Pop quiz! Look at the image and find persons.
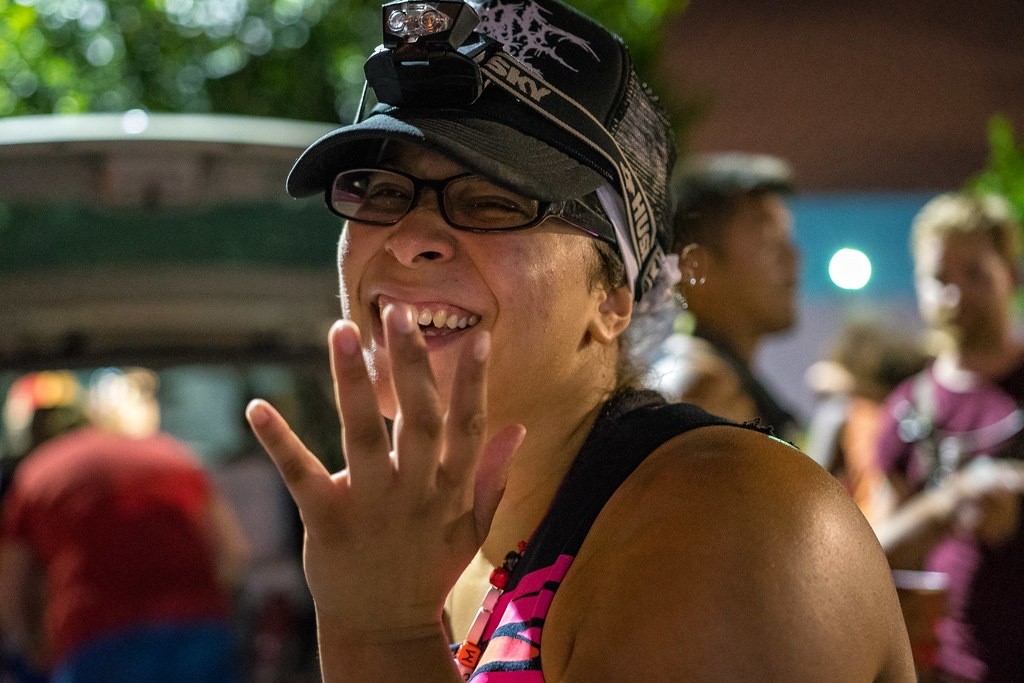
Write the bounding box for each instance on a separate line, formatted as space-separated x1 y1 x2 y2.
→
245 0 917 683
866 190 1024 683
644 151 804 443
0 405 251 683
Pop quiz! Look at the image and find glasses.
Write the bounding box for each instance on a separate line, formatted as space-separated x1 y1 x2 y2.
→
323 167 617 245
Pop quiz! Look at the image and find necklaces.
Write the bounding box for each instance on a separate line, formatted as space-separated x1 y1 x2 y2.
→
453 540 530 682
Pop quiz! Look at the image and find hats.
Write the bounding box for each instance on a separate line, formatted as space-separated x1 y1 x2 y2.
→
285 0 675 250
